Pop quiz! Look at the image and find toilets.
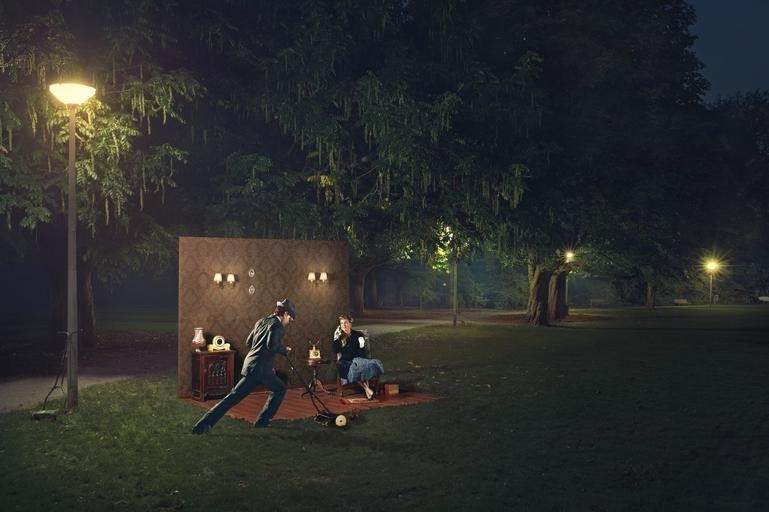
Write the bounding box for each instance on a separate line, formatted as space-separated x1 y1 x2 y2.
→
337 325 343 335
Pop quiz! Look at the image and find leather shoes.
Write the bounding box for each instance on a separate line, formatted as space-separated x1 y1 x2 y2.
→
333 325 342 341
358 337 365 349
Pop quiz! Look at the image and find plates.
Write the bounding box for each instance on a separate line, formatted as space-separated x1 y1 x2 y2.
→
193 349 236 401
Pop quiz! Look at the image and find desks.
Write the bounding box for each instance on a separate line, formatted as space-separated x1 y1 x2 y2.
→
705 259 719 311
49 79 98 410
565 252 573 305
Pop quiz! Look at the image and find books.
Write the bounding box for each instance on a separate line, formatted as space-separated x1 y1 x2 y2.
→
384 384 400 395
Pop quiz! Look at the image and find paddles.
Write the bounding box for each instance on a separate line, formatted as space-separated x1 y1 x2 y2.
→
181 382 442 424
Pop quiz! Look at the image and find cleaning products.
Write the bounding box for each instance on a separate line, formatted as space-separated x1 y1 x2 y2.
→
284 344 359 427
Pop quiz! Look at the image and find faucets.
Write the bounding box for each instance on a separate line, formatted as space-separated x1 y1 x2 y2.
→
365 387 374 400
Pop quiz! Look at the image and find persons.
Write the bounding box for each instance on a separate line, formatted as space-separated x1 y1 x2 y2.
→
191 298 297 435
332 313 385 401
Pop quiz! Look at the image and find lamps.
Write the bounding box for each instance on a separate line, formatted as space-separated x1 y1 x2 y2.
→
334 329 374 397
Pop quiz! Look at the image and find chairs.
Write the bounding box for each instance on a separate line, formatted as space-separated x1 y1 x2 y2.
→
255 419 272 428
192 424 205 434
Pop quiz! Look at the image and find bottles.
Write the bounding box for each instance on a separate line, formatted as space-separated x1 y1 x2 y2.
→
277 299 297 320
